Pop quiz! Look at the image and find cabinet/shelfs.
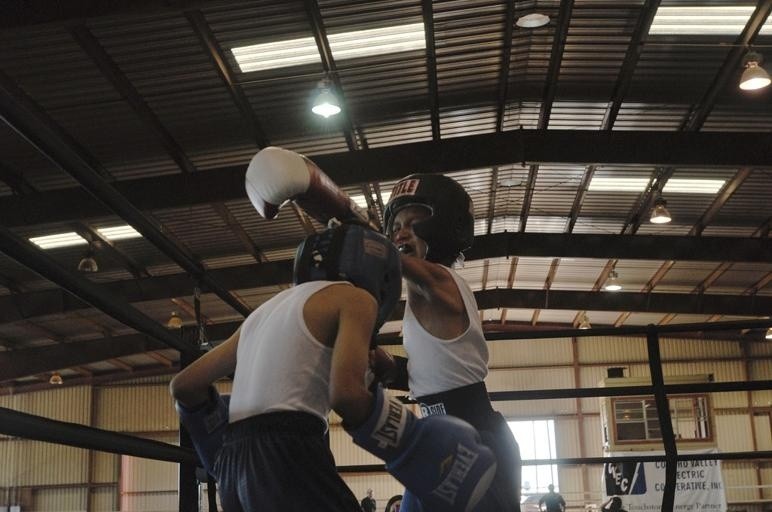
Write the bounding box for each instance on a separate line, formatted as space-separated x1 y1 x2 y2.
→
596 375 718 456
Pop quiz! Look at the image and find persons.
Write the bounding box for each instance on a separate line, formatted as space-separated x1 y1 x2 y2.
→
539 483 566 511
384 495 403 512
360 488 377 511
600 495 627 511
170 220 510 511
244 143 522 512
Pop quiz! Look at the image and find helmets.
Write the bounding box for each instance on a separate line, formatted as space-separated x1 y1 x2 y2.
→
291 223 402 350
382 172 475 267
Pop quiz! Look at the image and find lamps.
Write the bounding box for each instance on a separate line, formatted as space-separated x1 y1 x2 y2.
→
604 267 622 293
78 239 100 272
645 184 673 227
50 365 64 383
578 313 591 331
311 70 343 121
168 303 182 328
738 52 771 91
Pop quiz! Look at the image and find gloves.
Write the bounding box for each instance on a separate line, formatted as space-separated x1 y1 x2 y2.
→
244 145 370 228
340 381 498 511
174 387 229 480
